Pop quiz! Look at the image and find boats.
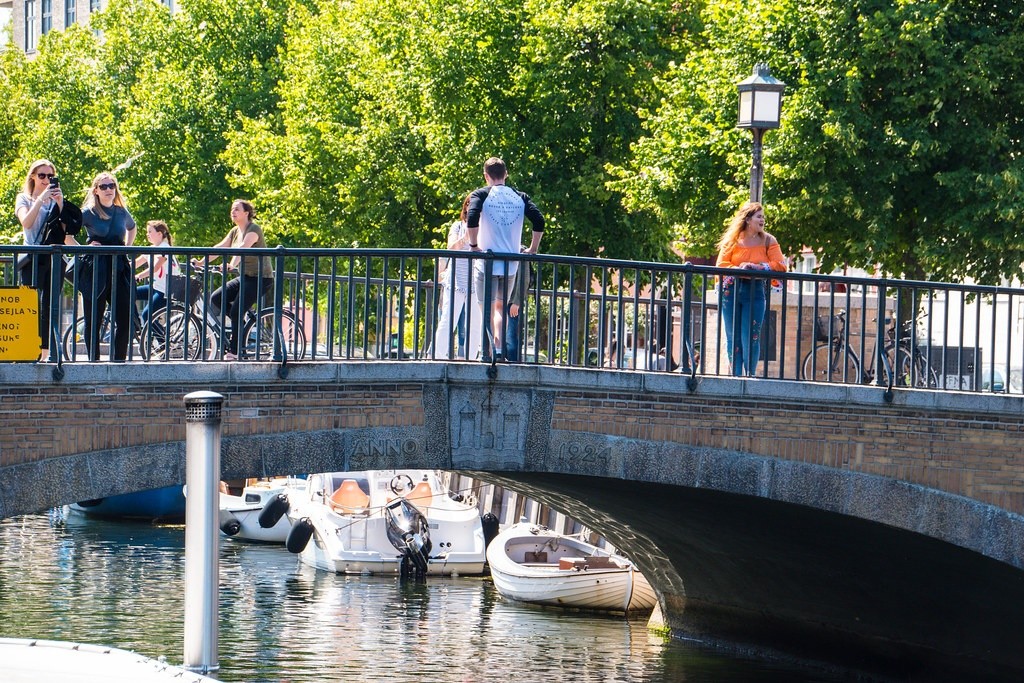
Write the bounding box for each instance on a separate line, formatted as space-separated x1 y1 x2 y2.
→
218 478 307 541
485 516 658 613
69 485 187 517
280 468 486 577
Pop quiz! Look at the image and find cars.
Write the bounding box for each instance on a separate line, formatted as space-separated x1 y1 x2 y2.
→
376 349 426 359
283 342 327 356
520 348 546 363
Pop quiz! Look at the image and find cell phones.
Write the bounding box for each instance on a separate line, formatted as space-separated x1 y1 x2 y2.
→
50 178 58 189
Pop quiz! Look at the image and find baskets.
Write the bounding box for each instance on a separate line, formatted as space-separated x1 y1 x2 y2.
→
817 314 838 341
887 326 921 347
166 273 202 303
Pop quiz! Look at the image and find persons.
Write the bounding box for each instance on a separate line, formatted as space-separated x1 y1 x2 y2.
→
64 172 136 362
426 157 546 363
129 221 182 361
190 198 274 361
603 337 699 375
715 201 786 378
15 159 66 363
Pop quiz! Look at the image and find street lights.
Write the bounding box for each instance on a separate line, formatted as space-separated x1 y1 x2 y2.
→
735 62 787 205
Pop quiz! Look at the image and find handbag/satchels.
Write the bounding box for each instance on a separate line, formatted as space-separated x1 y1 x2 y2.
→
65 254 106 300
14 253 31 272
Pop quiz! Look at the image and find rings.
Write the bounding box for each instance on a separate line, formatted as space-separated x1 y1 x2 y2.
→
47 190 49 192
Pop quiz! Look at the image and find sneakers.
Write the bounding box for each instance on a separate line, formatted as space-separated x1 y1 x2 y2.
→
103 335 112 342
224 353 238 360
492 348 505 361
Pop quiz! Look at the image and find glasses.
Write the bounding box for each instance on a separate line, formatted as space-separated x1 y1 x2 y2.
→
98 183 115 190
37 173 55 180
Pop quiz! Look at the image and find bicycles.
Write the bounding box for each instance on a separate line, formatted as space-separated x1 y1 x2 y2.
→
63 278 218 363
139 262 306 362
884 314 939 389
802 311 919 389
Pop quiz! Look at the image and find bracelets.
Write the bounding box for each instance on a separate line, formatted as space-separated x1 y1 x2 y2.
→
36 195 45 203
469 243 478 248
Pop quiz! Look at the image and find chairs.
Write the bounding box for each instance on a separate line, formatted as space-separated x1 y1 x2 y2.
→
331 479 370 516
387 482 433 514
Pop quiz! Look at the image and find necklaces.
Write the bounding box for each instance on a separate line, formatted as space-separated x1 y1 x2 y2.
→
32 196 49 205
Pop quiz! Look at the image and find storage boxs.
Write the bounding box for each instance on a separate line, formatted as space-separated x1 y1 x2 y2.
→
559 557 586 570
